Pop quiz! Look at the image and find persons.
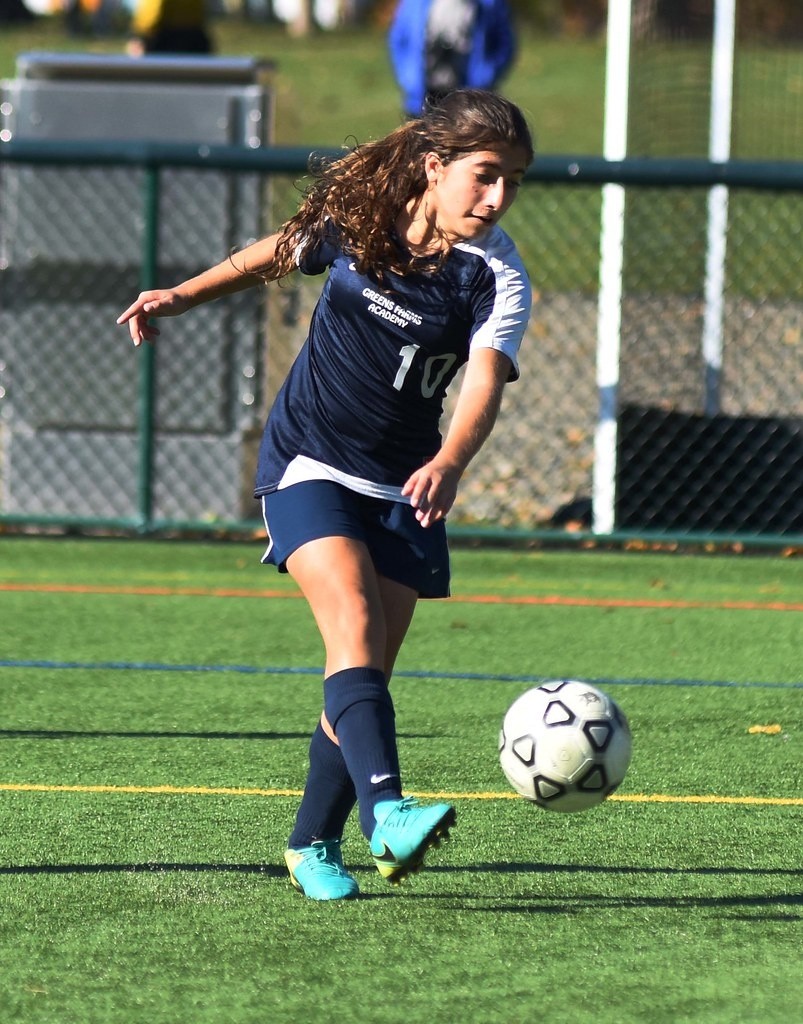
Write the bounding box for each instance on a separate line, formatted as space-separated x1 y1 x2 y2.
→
64 0 365 58
115 90 534 904
388 0 516 118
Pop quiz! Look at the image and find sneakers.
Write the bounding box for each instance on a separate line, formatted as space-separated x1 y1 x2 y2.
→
369 796 457 888
284 837 359 901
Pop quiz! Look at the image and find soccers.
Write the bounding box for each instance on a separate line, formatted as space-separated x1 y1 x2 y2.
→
498 679 635 814
